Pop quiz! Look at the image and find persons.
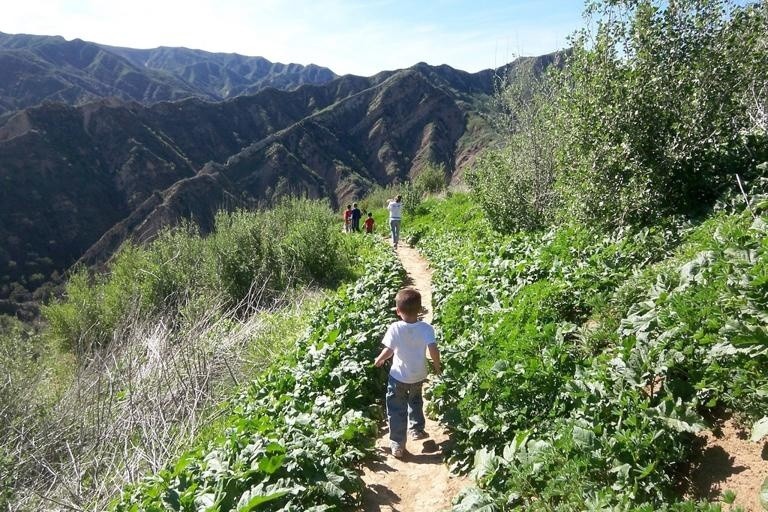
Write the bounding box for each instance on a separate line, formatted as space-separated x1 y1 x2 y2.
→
348 204 361 233
361 212 375 234
344 204 352 233
373 289 442 460
387 195 402 250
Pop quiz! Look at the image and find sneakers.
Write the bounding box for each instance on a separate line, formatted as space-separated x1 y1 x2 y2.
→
411 428 430 441
390 439 406 458
394 243 397 250
